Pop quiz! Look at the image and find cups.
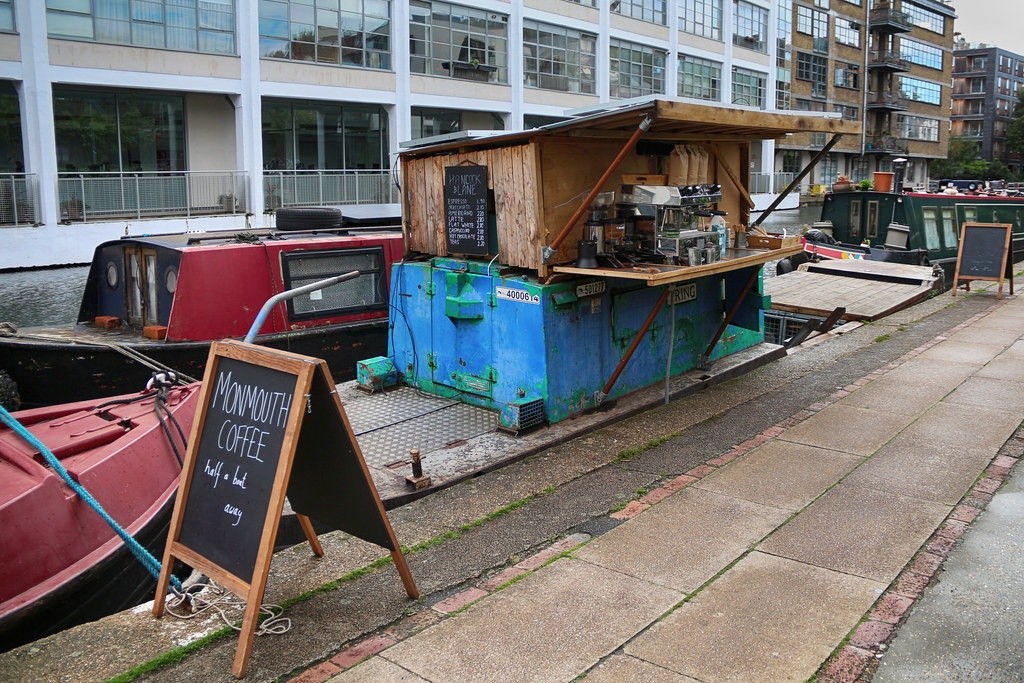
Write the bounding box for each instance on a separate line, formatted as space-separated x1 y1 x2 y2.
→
688 246 702 266
734 232 750 248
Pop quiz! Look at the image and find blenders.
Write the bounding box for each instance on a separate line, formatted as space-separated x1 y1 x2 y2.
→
582 191 624 256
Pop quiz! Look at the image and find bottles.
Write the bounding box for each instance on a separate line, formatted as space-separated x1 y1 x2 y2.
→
706 249 716 264
708 215 725 232
859 242 870 248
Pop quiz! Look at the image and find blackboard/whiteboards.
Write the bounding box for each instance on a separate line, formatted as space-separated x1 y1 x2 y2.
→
149 337 398 597
952 221 1014 282
443 164 490 258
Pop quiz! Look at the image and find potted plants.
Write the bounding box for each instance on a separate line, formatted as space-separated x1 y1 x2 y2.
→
265 183 280 206
860 179 873 191
217 193 237 210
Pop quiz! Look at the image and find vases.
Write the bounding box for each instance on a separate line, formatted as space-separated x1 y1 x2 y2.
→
872 172 895 192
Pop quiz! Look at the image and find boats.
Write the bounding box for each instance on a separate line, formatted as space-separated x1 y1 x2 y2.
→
0 206 404 415
777 157 1024 290
0 379 205 655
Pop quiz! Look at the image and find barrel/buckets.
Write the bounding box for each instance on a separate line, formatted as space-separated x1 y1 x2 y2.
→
812 220 833 239
884 223 911 249
872 171 895 192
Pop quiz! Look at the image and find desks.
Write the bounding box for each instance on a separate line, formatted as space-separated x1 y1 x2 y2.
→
441 61 498 82
